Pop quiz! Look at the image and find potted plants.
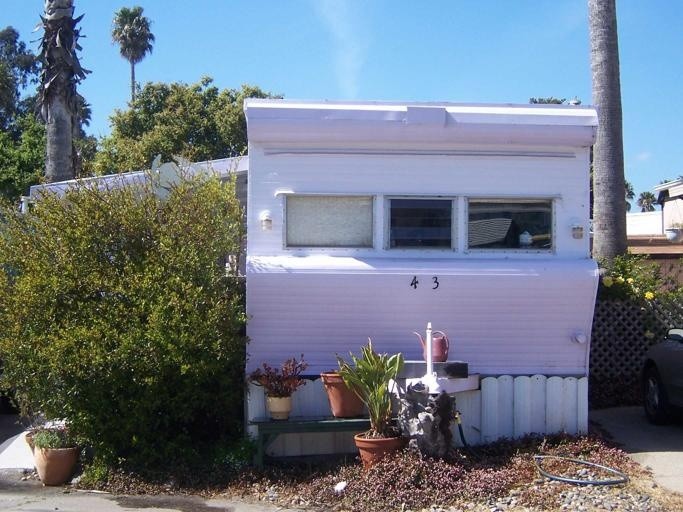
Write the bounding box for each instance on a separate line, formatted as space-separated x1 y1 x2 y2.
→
25 417 60 452
335 335 403 466
34 430 78 486
245 353 309 420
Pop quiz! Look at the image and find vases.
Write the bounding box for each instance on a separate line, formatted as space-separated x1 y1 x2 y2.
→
320 372 364 418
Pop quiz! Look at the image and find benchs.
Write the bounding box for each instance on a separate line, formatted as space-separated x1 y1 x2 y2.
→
249 416 399 471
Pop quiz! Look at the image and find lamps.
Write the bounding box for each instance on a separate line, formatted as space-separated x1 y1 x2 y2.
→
261 211 272 232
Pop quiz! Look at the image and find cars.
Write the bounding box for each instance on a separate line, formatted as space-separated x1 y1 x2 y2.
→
642 329 682 425
